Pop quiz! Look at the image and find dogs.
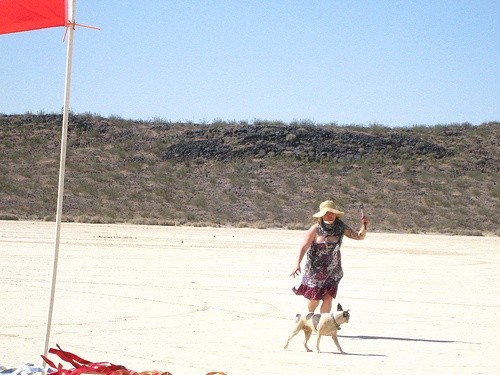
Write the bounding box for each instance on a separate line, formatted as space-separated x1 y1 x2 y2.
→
283 304 352 354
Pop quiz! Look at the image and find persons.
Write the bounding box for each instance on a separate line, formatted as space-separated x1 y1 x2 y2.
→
289 201 369 334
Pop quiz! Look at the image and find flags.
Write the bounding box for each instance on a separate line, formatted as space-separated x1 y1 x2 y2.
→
0 0 71 36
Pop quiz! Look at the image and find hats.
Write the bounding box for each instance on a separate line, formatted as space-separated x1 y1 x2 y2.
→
312 200 344 218
